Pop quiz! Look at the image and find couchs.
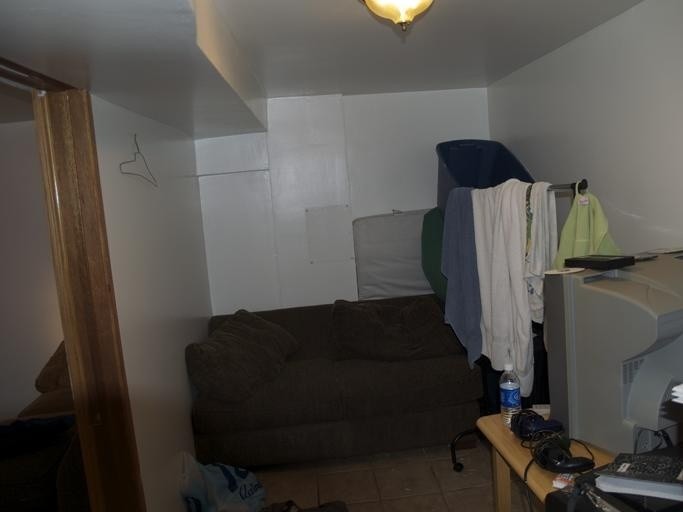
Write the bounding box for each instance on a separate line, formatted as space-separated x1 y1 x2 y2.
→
191 294 484 469
0 342 90 510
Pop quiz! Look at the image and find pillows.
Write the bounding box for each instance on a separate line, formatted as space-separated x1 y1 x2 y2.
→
329 300 450 361
185 309 300 403
0 414 75 460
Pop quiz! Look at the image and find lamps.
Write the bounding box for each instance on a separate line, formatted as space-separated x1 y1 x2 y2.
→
357 1 435 31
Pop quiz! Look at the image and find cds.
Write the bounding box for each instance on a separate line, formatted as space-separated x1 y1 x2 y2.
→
545 267 584 274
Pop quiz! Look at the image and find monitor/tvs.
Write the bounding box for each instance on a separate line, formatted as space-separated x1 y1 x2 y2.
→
543 248 683 455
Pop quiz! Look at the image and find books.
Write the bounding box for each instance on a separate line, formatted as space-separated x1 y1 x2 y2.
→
592 382 683 501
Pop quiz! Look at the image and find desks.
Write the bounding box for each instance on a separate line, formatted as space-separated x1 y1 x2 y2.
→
476 411 614 512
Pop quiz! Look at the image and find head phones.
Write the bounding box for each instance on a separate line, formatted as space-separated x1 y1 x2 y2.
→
511 411 564 439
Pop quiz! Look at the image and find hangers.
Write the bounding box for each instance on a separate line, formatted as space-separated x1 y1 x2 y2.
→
121 135 158 188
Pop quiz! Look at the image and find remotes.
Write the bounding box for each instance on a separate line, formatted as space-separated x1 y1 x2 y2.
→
532 405 550 414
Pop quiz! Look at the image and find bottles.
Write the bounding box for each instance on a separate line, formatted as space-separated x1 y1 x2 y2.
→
499 363 522 426
